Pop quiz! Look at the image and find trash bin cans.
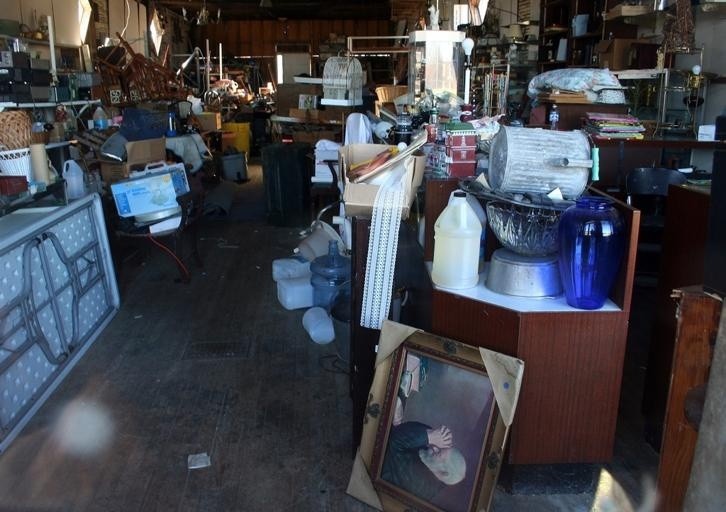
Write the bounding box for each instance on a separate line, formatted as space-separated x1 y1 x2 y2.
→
259 143 314 227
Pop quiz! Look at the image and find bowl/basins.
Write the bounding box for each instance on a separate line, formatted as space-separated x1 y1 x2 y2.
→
486 200 569 257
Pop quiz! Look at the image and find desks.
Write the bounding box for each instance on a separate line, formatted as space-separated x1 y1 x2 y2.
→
111 195 204 287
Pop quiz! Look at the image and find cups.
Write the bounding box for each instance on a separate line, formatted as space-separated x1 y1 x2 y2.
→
29 144 50 186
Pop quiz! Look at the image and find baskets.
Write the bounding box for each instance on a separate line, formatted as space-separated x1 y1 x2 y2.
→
1 110 35 150
374 84 409 103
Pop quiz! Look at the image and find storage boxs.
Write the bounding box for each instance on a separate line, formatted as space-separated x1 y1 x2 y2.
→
597 36 662 73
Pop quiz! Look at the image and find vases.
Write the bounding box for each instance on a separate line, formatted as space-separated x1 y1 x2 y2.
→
553 194 628 311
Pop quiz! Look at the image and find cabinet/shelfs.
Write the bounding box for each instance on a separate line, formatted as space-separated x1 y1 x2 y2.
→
0 31 101 177
535 0 586 82
0 188 123 458
470 39 538 126
628 178 715 390
339 209 425 462
406 29 470 106
529 95 667 210
404 168 646 502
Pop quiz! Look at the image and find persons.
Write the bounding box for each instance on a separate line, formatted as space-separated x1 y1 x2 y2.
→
377 413 472 504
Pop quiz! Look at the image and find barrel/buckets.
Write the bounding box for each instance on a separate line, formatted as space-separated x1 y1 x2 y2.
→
487 124 601 200
431 191 484 291
61 159 84 200
327 278 352 373
310 239 351 310
92 104 108 130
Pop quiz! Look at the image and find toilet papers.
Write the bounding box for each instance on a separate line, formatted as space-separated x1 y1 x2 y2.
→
29 143 60 188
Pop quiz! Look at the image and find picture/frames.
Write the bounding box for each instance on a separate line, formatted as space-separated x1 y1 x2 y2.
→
342 313 526 512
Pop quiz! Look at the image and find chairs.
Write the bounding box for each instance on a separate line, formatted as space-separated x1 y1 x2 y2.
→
624 165 687 234
97 29 190 103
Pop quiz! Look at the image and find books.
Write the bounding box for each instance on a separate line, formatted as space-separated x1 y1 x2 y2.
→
352 129 429 184
582 111 647 141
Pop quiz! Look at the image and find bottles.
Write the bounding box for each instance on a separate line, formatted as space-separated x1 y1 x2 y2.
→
38 14 49 40
548 103 559 130
428 106 440 126
92 107 109 129
165 112 177 136
557 197 620 309
394 112 413 144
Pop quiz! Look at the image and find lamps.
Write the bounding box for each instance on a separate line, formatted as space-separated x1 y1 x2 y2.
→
181 0 223 29
